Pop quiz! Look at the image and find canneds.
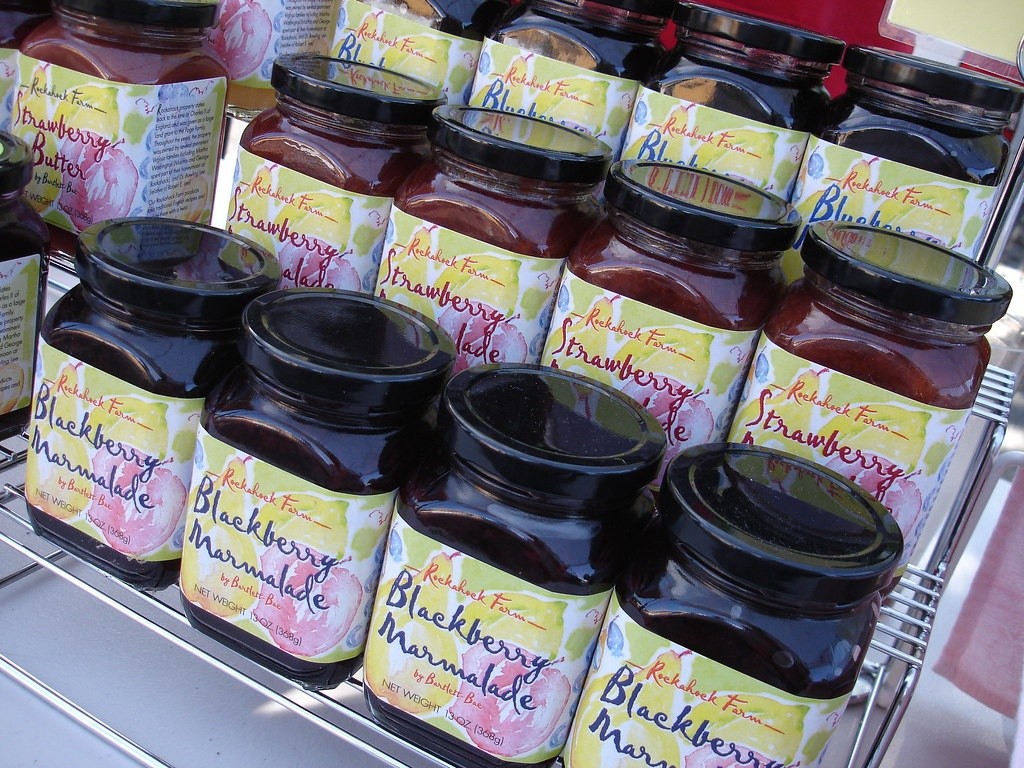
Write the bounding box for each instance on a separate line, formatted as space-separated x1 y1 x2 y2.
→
0 0 1024 768
